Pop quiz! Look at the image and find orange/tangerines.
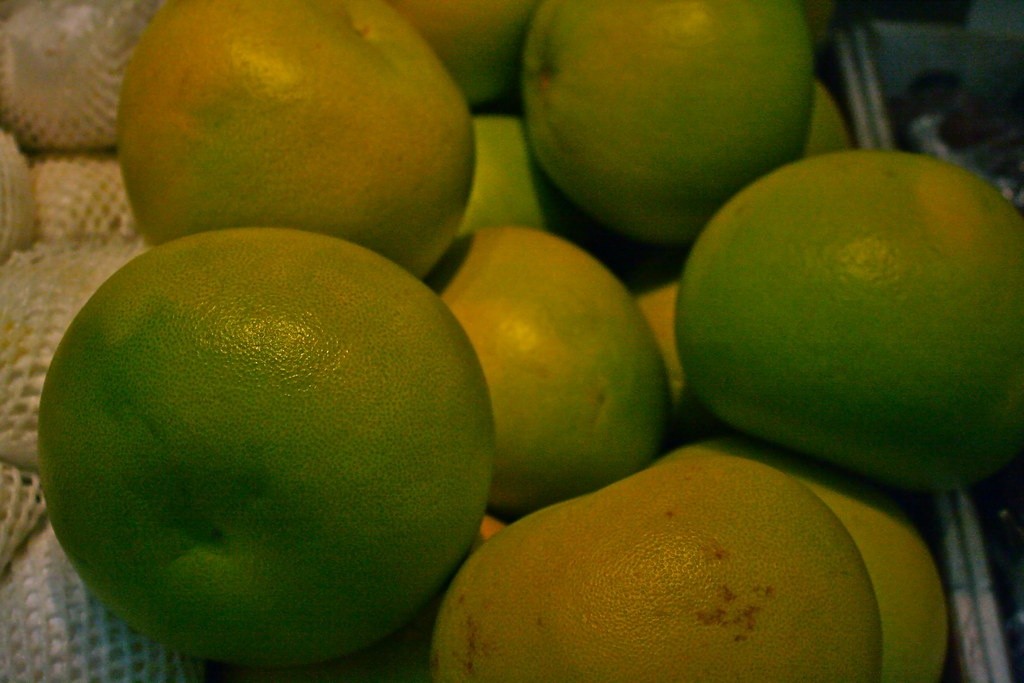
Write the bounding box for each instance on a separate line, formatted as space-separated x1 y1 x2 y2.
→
35 0 1024 683
